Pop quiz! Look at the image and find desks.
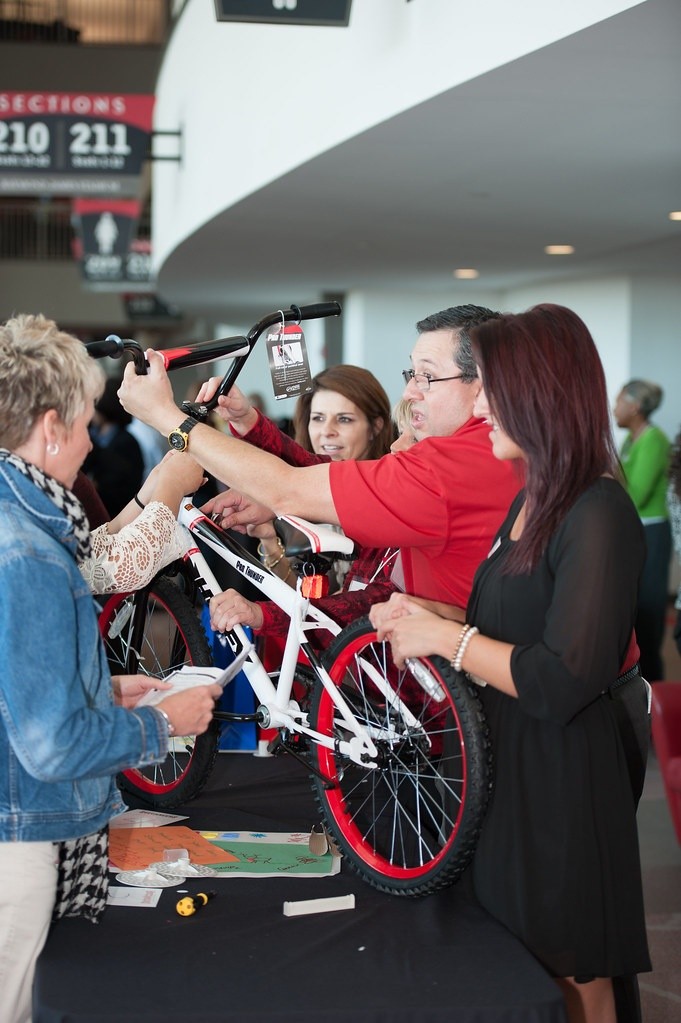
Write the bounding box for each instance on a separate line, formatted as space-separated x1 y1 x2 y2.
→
30 745 573 1023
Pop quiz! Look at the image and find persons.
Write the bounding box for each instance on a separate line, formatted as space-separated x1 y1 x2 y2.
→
0 311 222 1021
117 304 530 838
193 363 448 866
77 447 208 596
367 305 653 1023
612 378 676 684
69 374 145 534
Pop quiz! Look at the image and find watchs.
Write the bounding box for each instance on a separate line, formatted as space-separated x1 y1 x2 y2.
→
167 416 198 453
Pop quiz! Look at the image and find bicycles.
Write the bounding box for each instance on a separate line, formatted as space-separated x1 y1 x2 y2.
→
76 302 498 900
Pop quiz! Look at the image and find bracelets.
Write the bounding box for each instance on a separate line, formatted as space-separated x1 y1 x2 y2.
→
134 494 144 509
153 704 176 738
257 537 292 582
450 624 479 673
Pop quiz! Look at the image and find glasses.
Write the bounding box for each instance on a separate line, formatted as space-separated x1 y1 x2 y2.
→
402 370 479 392
